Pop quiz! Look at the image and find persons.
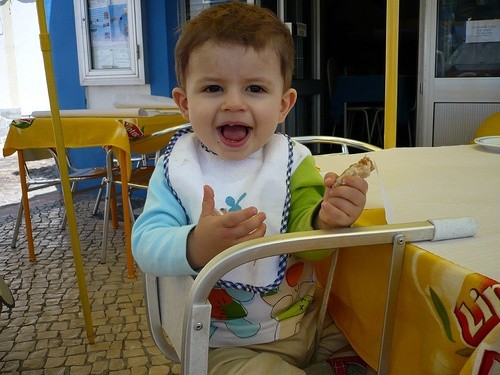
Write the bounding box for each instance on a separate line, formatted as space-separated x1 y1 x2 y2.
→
131 2 377 374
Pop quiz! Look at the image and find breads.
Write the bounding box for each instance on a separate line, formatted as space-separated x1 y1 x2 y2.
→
331 155 375 188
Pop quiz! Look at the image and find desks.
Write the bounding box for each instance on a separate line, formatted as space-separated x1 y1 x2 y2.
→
3 107 189 279
311 141 500 375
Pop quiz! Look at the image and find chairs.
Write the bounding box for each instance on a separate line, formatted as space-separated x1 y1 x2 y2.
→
11 147 121 248
101 123 192 264
145 135 477 374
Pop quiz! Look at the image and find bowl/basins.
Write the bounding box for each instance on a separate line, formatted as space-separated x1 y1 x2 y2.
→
474 135 500 153
